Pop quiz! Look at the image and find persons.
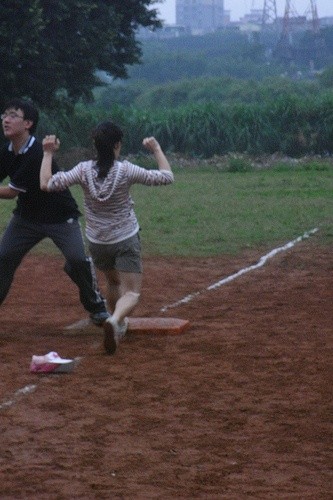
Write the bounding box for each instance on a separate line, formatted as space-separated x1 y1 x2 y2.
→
40 122 175 354
0 103 112 326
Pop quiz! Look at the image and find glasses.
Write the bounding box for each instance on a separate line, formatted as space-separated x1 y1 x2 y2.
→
1 113 26 121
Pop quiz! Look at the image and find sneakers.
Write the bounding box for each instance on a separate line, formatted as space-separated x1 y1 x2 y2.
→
89 311 110 326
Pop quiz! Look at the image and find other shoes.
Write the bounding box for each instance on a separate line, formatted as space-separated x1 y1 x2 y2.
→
103 317 119 353
115 316 128 338
